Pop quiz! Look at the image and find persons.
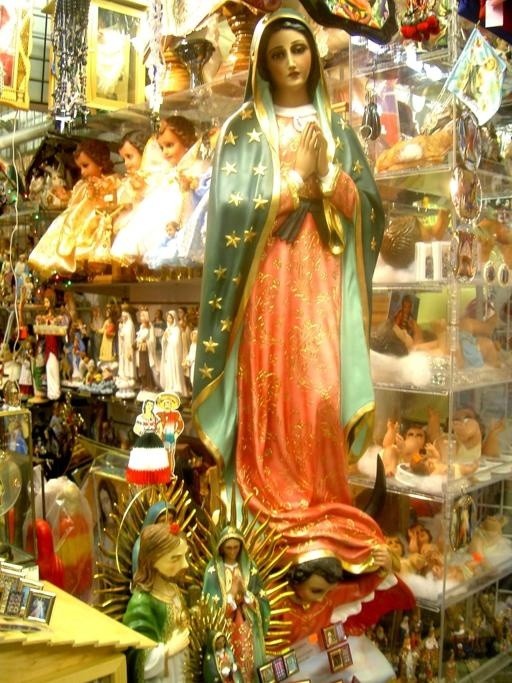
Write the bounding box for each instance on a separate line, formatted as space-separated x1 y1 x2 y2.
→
0 0 510 683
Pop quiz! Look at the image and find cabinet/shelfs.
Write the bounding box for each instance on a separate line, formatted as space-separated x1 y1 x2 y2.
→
1 0 511 683
